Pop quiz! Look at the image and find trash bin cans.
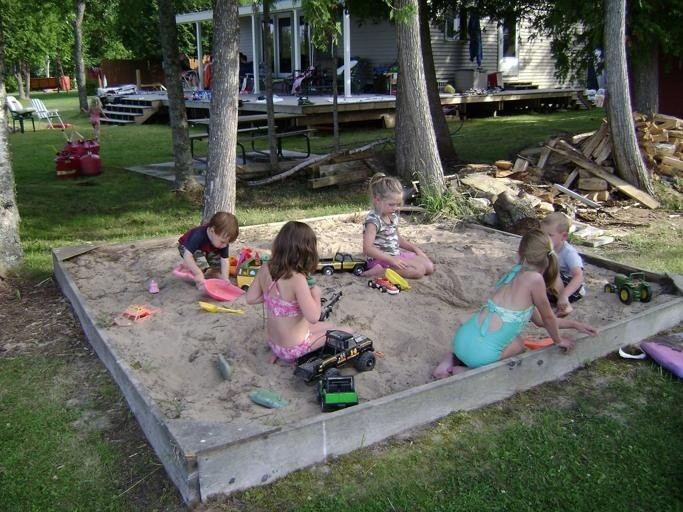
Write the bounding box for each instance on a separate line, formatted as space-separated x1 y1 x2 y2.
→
453 68 488 93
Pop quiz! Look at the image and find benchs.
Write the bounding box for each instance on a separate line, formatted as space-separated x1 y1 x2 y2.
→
189 126 277 141
238 129 316 142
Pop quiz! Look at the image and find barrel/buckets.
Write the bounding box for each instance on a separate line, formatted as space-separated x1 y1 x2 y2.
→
443 84 455 94
52 137 101 180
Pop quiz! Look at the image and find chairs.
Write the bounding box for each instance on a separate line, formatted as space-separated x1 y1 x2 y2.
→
239 64 265 100
32 99 65 129
162 61 194 93
4 96 36 133
281 57 360 96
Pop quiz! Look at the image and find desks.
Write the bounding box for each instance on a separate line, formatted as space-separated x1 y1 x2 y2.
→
187 113 310 163
382 73 397 95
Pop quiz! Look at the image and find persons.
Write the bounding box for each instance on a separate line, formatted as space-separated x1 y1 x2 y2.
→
539 210 587 319
80 95 109 145
429 227 598 384
358 171 434 280
244 219 345 365
176 212 239 285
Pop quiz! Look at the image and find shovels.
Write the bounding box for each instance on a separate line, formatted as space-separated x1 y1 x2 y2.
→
172 264 247 314
